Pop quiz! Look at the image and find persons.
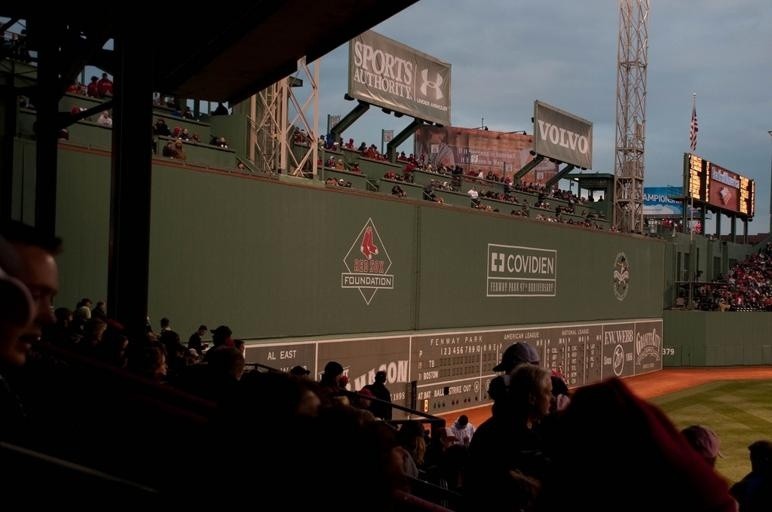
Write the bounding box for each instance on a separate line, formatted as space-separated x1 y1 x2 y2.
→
469 169 619 232
289 125 385 188
416 123 456 172
1 67 248 174
430 340 771 511
385 151 453 204
2 217 430 509
450 176 463 193
668 241 771 312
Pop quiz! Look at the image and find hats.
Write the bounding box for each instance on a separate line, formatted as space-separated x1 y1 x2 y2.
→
492 341 541 373
679 423 726 461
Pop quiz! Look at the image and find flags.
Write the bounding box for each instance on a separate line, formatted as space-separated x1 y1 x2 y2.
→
689 104 699 152
